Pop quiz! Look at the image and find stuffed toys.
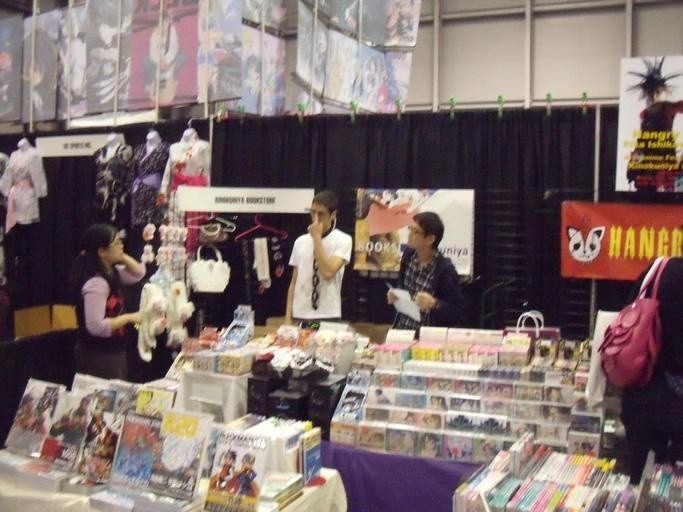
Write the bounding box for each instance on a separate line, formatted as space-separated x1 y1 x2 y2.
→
167 282 195 351
135 282 170 363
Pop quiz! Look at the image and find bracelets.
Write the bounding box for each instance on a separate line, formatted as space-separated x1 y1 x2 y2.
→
431 298 440 312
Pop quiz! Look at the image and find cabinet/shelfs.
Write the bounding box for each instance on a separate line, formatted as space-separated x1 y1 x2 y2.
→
347 276 518 328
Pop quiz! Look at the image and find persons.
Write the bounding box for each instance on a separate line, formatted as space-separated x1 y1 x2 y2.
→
620 255 683 484
94 133 133 223
0 138 48 229
285 192 353 328
624 56 683 194
130 130 169 225
70 224 145 379
386 212 464 331
144 12 186 92
159 128 212 254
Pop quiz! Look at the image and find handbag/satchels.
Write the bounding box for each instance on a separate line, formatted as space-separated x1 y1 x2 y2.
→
502 327 560 342
185 260 233 295
594 293 665 385
195 222 230 246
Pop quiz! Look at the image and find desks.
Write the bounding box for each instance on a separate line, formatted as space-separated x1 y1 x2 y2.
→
0 448 348 512
322 440 483 512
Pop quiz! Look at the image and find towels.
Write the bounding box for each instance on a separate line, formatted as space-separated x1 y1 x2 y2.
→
243 236 285 294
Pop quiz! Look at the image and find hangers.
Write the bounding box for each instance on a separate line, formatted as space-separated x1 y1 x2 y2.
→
234 215 289 240
183 213 236 234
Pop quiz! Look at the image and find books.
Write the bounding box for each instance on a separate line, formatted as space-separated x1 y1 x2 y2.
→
0 0 420 112
3 375 320 511
329 327 683 512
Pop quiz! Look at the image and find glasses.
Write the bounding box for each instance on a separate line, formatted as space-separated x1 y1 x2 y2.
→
406 225 427 235
304 207 331 218
